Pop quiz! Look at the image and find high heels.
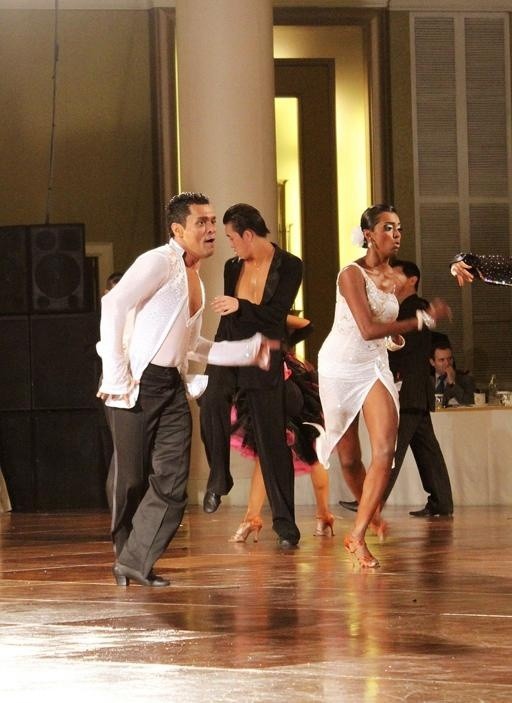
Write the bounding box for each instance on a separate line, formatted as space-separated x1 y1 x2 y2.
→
368 512 388 541
344 534 380 568
227 519 262 543
313 513 335 536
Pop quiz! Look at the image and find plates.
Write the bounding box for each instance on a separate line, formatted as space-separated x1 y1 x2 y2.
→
472 404 488 408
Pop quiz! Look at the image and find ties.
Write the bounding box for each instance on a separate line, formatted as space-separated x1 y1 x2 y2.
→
436 375 446 394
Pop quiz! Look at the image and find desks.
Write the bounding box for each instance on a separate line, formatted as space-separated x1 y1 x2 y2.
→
427 405 511 506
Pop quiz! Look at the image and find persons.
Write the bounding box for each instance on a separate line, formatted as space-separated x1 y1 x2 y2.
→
98 191 283 589
315 201 455 571
196 201 305 548
426 340 478 407
103 271 125 295
339 259 453 517
230 307 335 543
449 251 511 288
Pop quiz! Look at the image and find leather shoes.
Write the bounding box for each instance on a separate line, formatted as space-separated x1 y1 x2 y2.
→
276 538 295 548
111 559 169 586
409 509 452 516
203 491 220 513
339 501 357 511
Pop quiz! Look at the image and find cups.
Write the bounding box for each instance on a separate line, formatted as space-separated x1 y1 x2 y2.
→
473 393 487 406
434 394 443 409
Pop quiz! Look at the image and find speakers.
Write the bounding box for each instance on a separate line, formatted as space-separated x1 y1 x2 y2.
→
0 412 34 513
28 223 85 315
31 317 99 411
0 316 32 412
0 224 31 313
35 411 102 513
